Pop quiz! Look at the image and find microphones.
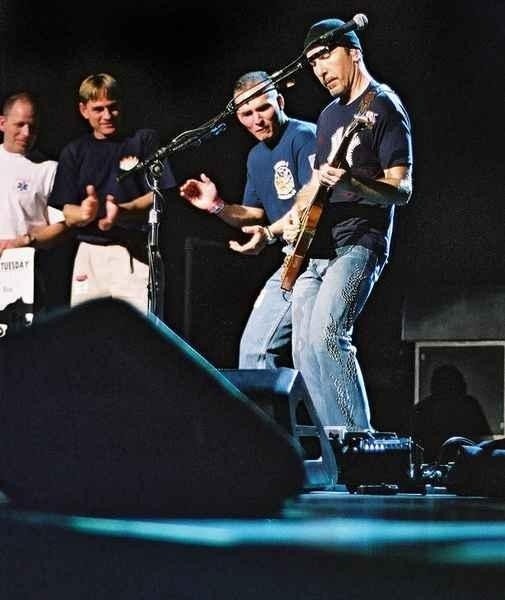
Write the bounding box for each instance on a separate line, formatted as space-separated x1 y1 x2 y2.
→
318 12 368 45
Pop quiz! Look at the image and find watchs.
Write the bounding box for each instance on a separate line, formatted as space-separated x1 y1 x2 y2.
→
263 225 278 246
23 233 36 246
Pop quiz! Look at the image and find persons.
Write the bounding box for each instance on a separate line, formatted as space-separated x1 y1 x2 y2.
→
178 70 317 421
238 17 414 491
48 72 178 315
0 92 68 333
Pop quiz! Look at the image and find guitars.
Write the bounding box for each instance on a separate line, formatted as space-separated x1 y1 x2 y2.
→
280 90 379 293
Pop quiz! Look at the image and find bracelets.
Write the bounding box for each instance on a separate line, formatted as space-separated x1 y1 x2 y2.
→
211 200 224 218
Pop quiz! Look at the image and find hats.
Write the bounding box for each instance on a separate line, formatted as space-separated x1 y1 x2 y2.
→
304 18 363 52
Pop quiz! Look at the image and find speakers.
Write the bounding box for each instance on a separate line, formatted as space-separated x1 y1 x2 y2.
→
203 368 335 494
412 341 504 488
0 296 305 519
